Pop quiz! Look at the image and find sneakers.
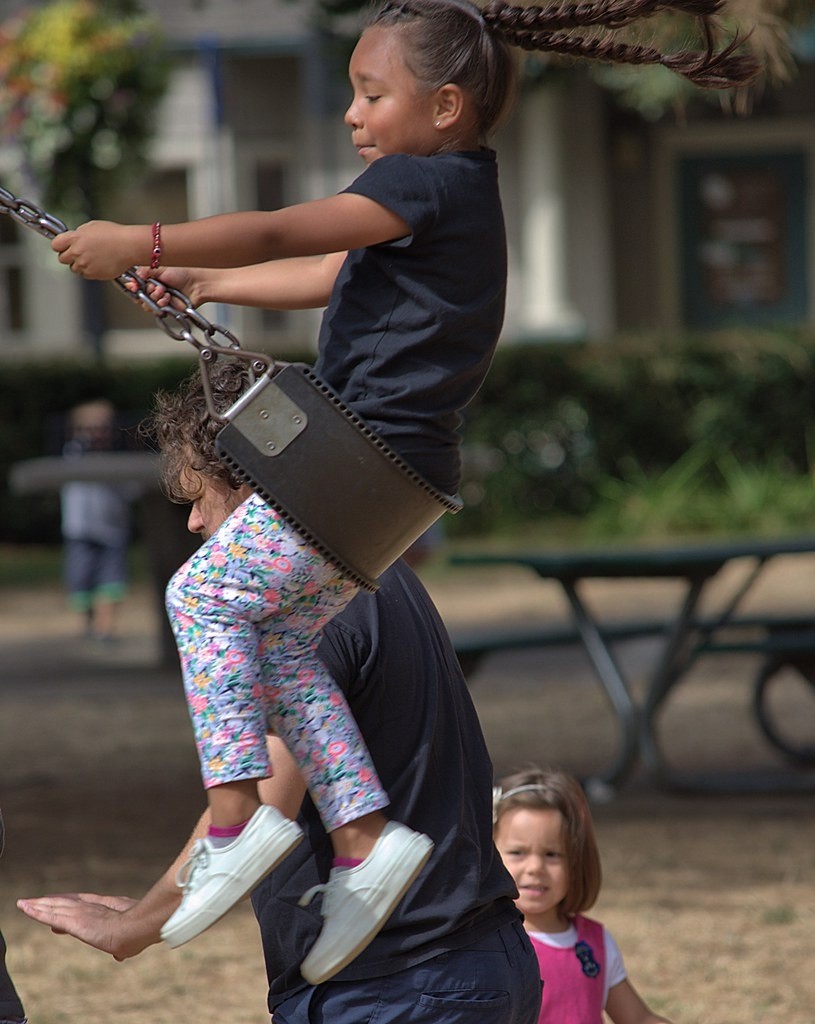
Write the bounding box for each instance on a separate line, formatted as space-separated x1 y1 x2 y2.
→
299 821 435 985
159 803 304 950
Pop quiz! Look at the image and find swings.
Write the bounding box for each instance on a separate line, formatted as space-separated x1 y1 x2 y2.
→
0 181 467 598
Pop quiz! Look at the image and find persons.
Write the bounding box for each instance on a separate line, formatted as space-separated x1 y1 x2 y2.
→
492 770 670 1023
17 363 543 1024
52 0 763 982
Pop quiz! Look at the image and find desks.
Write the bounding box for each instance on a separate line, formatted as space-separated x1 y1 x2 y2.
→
442 539 814 798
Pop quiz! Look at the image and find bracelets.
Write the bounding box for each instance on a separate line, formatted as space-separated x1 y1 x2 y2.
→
151 222 162 269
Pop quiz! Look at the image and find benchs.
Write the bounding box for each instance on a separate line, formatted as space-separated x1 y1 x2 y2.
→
444 607 815 798
